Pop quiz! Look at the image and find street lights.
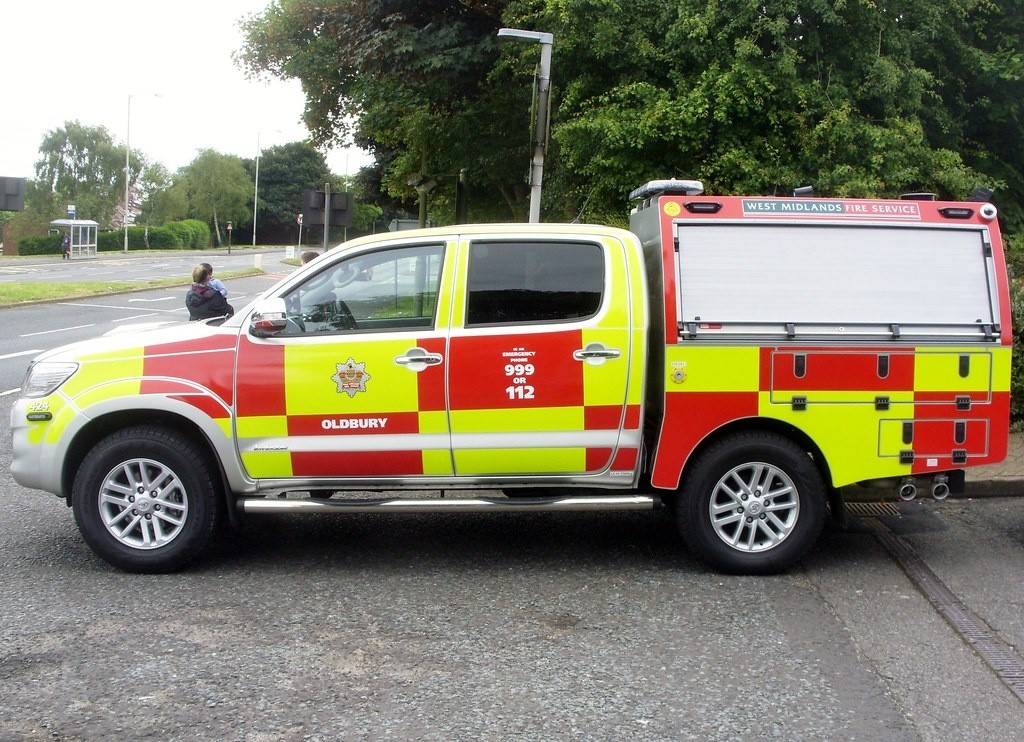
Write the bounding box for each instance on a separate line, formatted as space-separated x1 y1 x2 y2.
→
253 128 283 247
495 27 553 224
124 93 164 252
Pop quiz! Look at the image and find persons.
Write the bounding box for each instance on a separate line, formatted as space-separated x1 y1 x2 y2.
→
185 265 234 322
201 263 227 298
301 252 320 268
62 232 71 259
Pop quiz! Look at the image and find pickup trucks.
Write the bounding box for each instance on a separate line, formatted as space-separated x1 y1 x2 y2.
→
8 177 1012 578
